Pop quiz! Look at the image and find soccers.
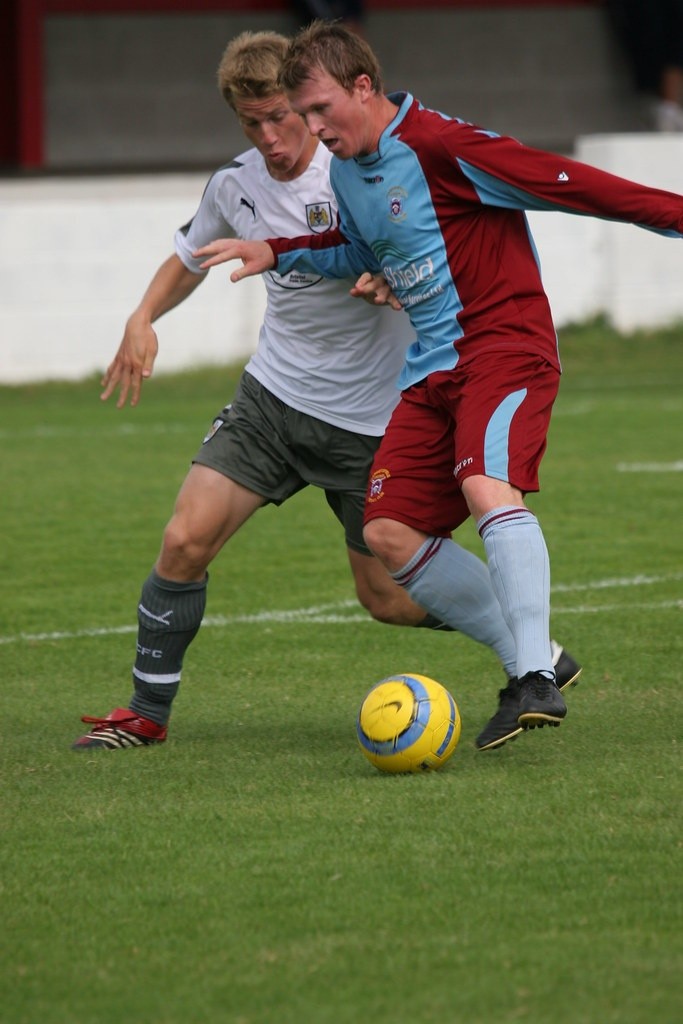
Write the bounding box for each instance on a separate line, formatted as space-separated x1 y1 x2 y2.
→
352 670 466 782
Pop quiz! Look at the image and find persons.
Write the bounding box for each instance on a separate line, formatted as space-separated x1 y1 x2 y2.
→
192 18 682 750
651 67 682 133
70 29 581 750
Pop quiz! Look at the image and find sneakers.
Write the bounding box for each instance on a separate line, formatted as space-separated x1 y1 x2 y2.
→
552 647 582 692
517 670 567 730
73 708 168 750
477 676 524 751
